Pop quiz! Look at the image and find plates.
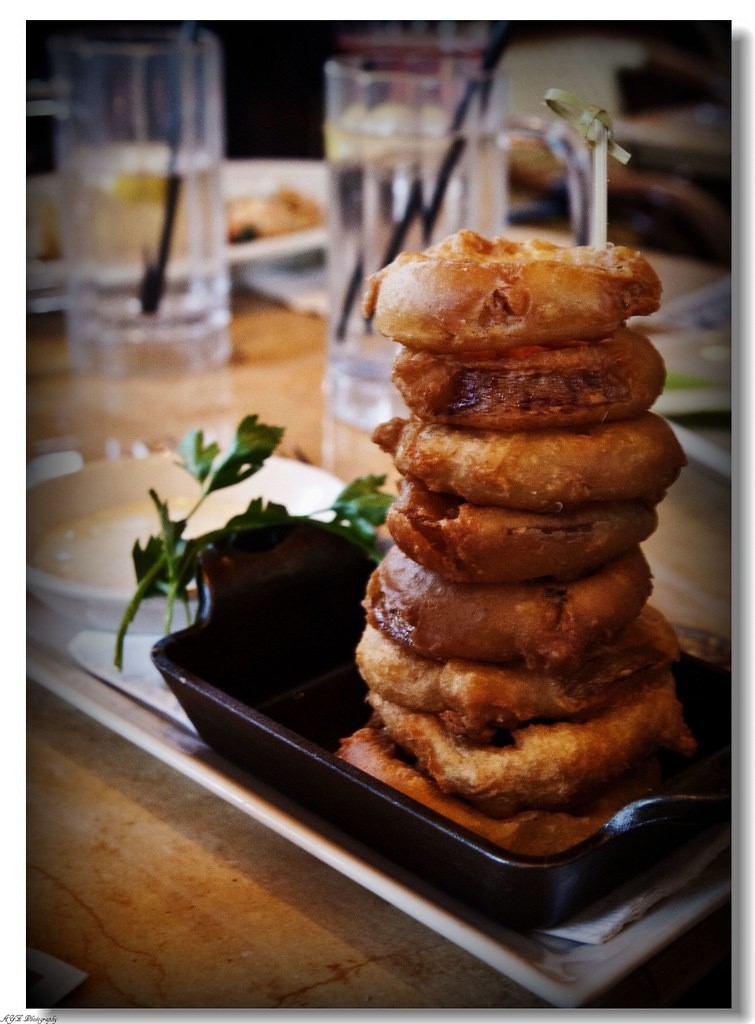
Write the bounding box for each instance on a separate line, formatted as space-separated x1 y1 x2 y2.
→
26 160 328 288
26 609 732 1007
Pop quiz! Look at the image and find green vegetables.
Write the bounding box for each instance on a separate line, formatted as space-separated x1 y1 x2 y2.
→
113 414 394 670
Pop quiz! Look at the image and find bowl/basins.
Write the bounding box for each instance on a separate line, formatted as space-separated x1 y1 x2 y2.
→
151 523 732 929
26 454 357 636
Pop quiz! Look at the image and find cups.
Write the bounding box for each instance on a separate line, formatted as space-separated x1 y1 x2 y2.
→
327 57 509 429
49 29 233 379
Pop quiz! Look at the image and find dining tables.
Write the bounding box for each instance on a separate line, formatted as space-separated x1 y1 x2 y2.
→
26 216 737 1008
